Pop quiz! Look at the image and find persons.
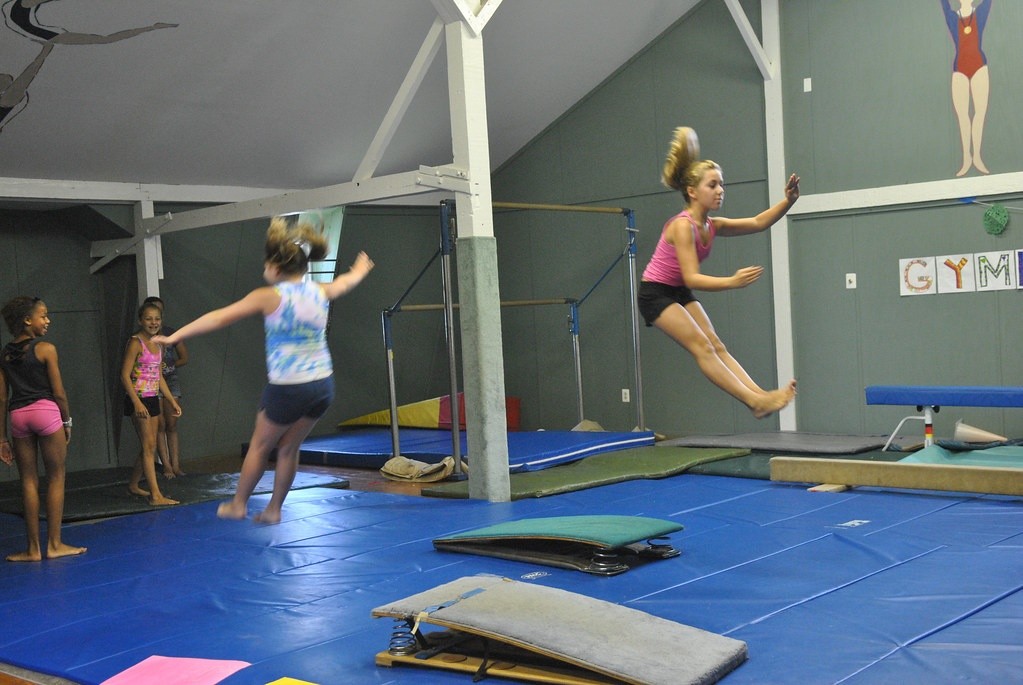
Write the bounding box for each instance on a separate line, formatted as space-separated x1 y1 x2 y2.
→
118 304 183 506
143 296 190 480
149 218 374 525
0 294 88 562
638 126 800 418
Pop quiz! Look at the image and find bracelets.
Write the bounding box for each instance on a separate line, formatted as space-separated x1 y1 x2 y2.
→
63 417 73 428
0 441 8 444
